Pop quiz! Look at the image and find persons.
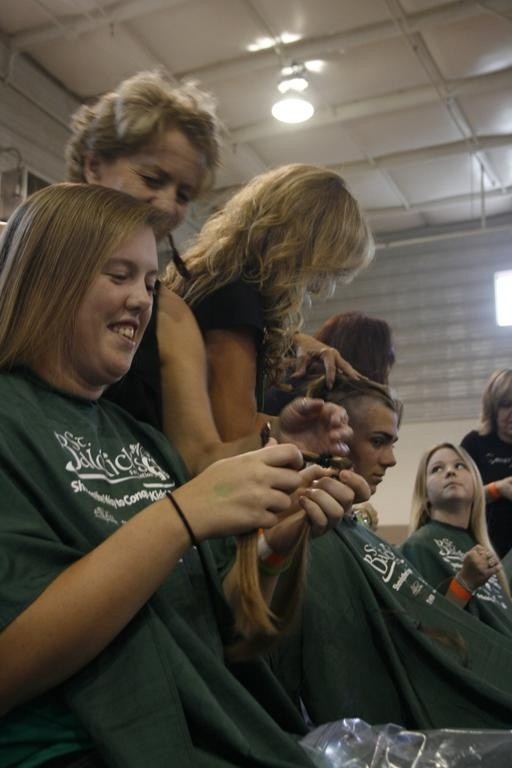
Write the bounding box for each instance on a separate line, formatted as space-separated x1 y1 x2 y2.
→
460 369 511 558
313 310 396 532
1 180 374 766
270 370 511 728
0 70 354 480
162 163 371 444
396 441 511 641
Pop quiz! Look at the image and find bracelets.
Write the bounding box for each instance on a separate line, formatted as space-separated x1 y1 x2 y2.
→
487 482 499 497
167 490 200 550
257 530 292 575
447 576 472 601
257 419 269 446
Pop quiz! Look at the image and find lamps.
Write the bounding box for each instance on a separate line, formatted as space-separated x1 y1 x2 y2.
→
269 62 318 126
